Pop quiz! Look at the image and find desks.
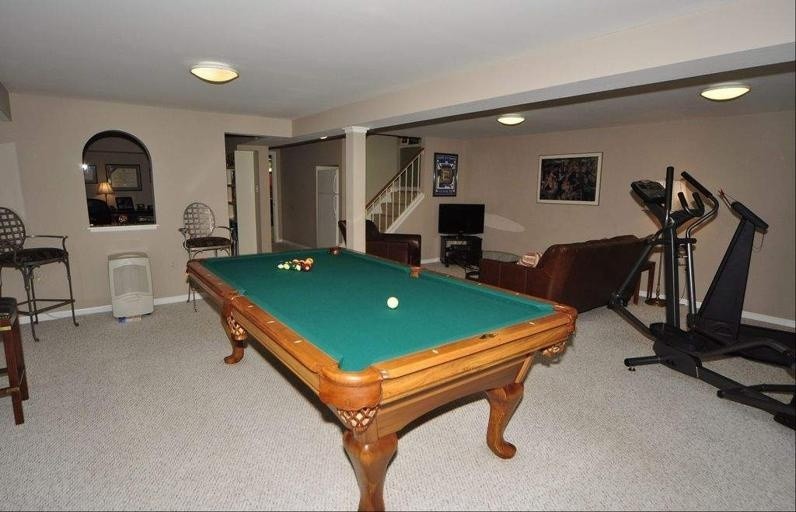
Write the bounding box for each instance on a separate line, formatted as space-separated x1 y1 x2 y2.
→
187 247 580 512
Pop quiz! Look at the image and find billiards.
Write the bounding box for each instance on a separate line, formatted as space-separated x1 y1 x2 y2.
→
277 258 313 271
387 296 398 309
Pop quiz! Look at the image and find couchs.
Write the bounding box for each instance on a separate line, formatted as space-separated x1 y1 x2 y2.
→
477 235 640 314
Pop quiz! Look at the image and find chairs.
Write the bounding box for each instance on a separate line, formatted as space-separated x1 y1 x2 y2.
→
178 201 235 312
337 217 423 269
0 207 79 342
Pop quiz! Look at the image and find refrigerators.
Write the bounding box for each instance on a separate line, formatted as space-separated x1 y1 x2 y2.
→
316 167 340 249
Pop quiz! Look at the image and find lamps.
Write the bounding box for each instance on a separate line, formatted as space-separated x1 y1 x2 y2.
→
96 182 114 204
187 61 241 84
496 112 525 127
699 81 751 102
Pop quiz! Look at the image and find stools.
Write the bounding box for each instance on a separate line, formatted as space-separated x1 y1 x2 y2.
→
0 296 29 425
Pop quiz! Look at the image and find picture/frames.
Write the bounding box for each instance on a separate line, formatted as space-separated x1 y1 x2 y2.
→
83 165 97 184
115 196 134 210
432 152 460 197
104 163 142 192
536 152 604 207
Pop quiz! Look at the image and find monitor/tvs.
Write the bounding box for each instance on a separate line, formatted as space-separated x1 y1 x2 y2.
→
439 204 484 240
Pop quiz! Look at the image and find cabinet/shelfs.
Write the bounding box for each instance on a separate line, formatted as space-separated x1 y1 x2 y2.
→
439 235 482 268
